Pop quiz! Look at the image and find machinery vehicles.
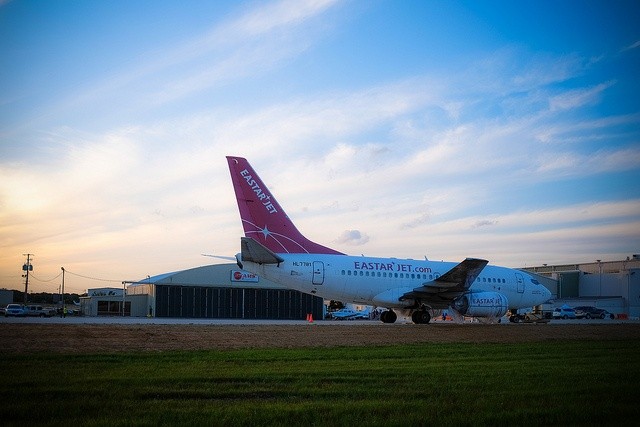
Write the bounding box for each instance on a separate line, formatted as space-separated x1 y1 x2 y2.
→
331 306 371 320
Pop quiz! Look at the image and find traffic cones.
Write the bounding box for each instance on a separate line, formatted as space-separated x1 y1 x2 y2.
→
306 313 309 320
309 313 313 322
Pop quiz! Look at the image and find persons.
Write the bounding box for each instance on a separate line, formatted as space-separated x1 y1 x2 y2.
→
63 305 68 318
372 309 375 317
375 309 378 317
149 305 152 318
59 306 63 317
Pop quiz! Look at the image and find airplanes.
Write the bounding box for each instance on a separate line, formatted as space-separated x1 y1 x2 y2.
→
227 156 552 324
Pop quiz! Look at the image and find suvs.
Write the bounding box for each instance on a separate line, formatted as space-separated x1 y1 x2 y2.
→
574 305 605 319
553 307 575 319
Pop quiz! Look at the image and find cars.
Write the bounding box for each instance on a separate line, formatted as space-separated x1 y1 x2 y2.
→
4 303 24 317
24 304 48 317
